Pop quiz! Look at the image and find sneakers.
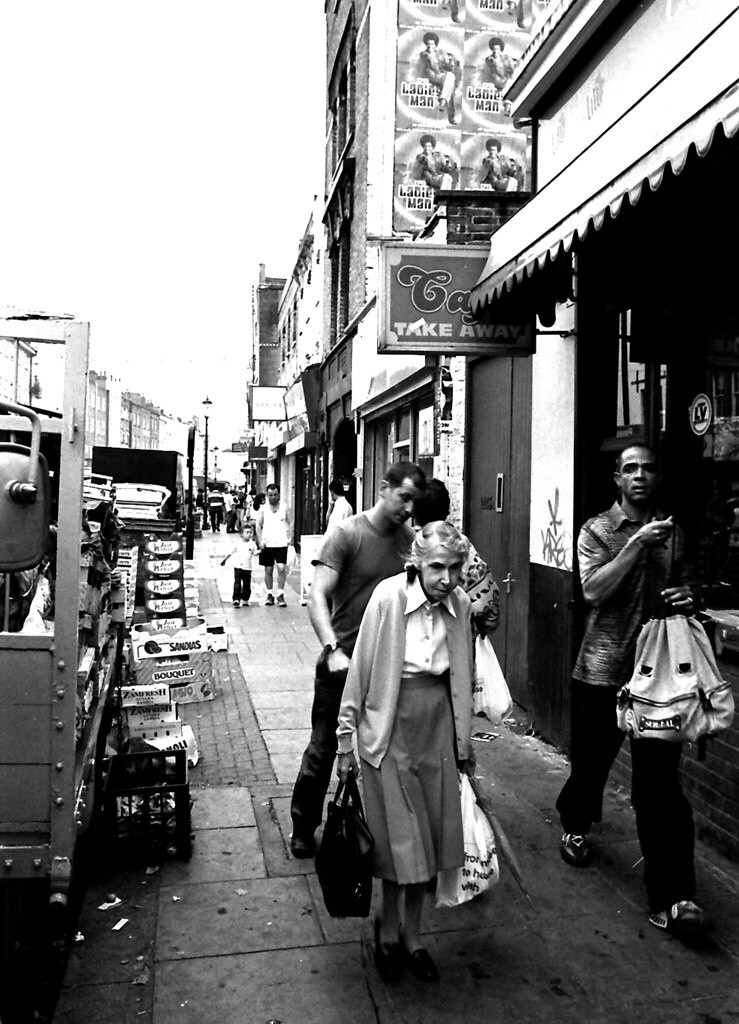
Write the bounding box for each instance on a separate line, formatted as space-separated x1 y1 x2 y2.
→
561 829 588 866
649 902 705 929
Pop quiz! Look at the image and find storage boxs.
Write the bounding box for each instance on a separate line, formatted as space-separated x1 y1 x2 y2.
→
95 535 213 872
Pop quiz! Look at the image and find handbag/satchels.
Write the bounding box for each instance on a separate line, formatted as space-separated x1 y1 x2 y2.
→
436 771 500 909
315 768 374 920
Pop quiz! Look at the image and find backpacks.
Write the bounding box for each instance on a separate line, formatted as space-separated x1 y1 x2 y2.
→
616 596 735 741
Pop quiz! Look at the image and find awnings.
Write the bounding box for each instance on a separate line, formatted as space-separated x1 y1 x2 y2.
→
467 7 739 328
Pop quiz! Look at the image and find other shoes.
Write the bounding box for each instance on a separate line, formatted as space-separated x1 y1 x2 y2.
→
374 918 404 980
277 594 287 607
227 530 240 533
233 600 240 605
265 594 274 605
290 827 316 857
242 598 248 606
402 929 437 979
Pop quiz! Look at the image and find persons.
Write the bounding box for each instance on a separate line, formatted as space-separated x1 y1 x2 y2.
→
334 521 477 977
485 38 515 116
291 460 426 860
506 0 525 28
419 32 456 126
197 485 265 532
416 134 455 190
220 525 261 606
555 438 705 928
410 480 499 637
327 478 352 532
255 484 294 606
441 0 460 22
480 139 524 191
